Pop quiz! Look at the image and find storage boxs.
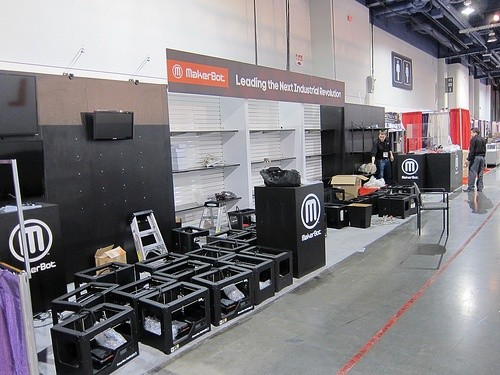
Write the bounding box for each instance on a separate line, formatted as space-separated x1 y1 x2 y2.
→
331 175 369 200
94 244 127 275
324 203 372 229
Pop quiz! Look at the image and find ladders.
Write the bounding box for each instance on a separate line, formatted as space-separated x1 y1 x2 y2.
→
130 209 169 262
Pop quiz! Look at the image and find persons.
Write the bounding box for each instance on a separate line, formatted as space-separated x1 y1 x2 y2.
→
463 127 486 192
371 130 394 185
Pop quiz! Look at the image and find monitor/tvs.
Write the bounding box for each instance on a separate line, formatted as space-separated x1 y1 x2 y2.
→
0 71 39 136
93 110 134 140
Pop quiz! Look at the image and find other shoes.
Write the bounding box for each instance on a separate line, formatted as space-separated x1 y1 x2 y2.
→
477 189 482 192
463 188 473 192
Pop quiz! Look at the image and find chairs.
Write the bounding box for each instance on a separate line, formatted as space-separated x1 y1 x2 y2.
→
415 183 449 237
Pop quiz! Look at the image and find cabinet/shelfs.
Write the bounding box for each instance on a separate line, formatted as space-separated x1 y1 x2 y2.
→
250 128 297 196
170 130 242 212
305 129 336 181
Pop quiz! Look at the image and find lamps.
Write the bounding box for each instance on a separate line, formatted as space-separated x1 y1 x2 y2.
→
461 0 474 15
482 49 492 62
487 30 497 42
